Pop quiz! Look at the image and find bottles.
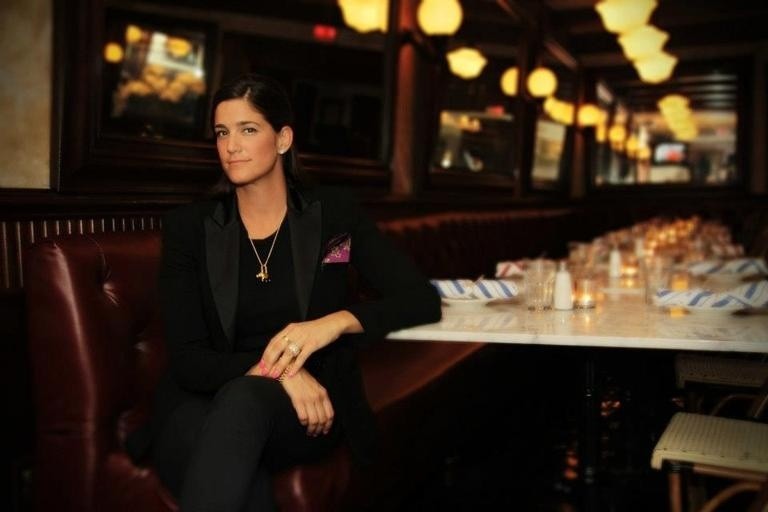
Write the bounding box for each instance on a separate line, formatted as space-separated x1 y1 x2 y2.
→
554 260 574 311
607 243 622 279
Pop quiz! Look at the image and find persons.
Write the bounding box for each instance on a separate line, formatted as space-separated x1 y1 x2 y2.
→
126 74 442 512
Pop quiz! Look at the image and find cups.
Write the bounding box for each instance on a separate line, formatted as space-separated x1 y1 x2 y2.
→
575 275 598 310
523 258 557 313
641 254 675 306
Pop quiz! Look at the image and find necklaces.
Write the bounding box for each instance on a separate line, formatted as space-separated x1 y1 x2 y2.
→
236 206 288 282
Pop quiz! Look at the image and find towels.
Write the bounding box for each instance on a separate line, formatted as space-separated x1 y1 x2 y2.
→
688 257 768 275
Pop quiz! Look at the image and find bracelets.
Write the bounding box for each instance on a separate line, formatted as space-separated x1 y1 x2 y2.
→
277 358 297 385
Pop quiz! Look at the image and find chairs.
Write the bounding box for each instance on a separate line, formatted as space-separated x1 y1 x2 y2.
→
650 351 768 512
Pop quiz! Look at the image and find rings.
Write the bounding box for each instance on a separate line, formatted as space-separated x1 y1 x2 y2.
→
283 336 290 345
289 344 301 356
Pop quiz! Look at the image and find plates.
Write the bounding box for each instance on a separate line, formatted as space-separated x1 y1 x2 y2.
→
684 306 741 319
440 296 496 311
